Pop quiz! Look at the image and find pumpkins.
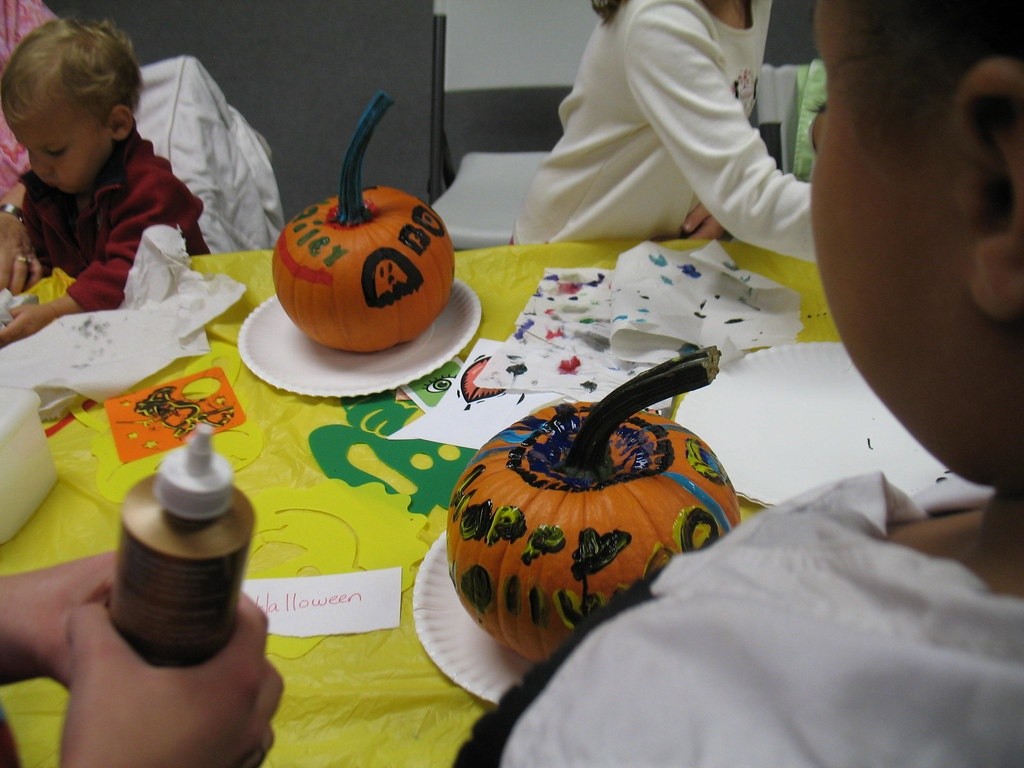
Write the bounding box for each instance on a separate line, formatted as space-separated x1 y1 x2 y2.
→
444 345 741 666
271 88 456 353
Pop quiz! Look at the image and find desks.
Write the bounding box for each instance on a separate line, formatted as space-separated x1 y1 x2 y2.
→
0 230 853 768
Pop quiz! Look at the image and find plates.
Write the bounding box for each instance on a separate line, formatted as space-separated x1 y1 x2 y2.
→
412 530 536 707
236 276 483 399
673 340 944 512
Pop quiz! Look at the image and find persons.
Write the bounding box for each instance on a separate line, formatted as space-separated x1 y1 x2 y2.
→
0 548 284 768
0 0 64 295
511 0 824 264
449 0 1024 768
0 14 212 348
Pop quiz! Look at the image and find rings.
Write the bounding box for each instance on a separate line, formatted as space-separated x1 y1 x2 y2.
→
16 253 31 263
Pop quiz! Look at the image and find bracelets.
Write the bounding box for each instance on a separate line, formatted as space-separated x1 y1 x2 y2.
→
0 203 25 224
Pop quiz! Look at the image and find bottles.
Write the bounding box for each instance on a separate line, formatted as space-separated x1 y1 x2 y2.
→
106 423 257 670
